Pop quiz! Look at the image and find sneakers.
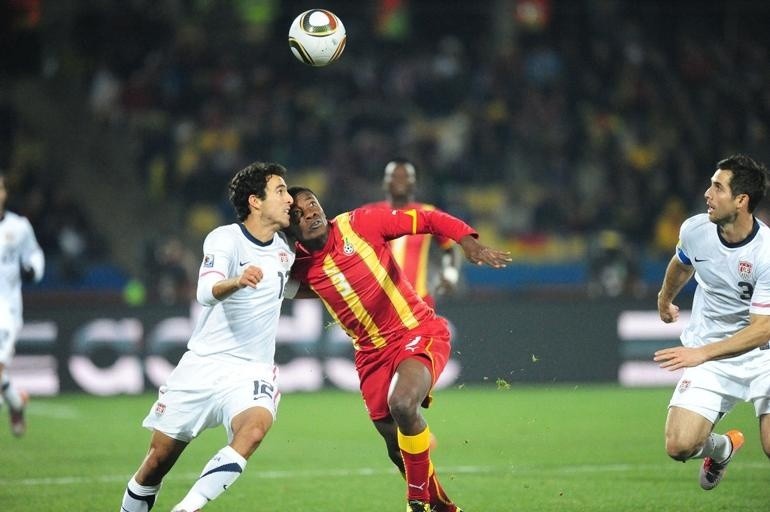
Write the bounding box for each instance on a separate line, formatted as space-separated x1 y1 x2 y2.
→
405 498 462 512
700 426 743 491
9 390 29 436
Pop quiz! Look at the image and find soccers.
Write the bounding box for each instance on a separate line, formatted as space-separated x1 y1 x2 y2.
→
288 8 347 66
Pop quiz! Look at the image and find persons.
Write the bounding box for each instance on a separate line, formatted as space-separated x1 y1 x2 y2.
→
649 151 770 494
1 174 50 441
280 181 518 512
115 160 325 512
